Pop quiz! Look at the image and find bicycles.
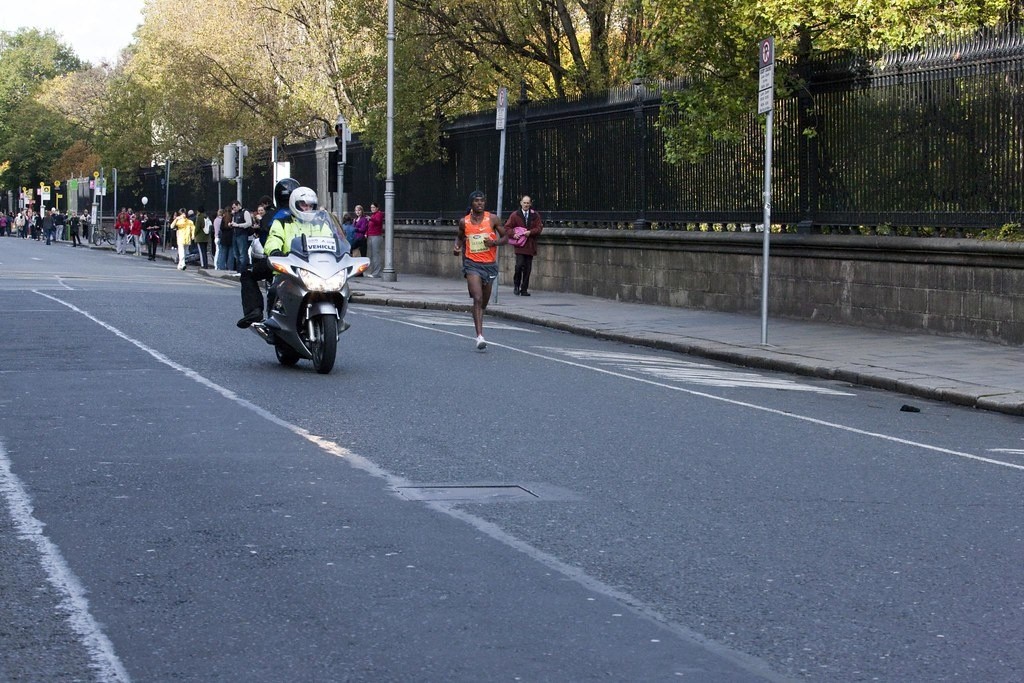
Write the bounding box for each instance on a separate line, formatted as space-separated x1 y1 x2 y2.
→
92 221 117 246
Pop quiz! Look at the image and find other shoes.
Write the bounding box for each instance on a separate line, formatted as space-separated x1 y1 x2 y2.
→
338 321 350 334
237 312 263 329
180 266 186 270
133 252 142 256
513 284 520 296
521 291 531 296
367 274 373 278
148 257 156 261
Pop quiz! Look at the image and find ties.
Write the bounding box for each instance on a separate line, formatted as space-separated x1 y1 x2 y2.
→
525 211 528 227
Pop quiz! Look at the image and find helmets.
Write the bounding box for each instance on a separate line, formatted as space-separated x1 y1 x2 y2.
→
275 178 301 208
289 186 318 221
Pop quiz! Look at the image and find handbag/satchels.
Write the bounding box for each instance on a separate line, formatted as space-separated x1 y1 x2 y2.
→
139 229 146 245
508 226 528 246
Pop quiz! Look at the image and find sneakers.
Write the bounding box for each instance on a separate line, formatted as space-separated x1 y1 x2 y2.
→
476 336 487 350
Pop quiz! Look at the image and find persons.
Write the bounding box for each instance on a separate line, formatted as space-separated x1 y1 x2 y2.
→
453 191 507 349
0 177 383 329
504 195 543 296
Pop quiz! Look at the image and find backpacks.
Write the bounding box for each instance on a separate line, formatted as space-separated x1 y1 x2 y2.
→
242 208 254 236
202 215 212 234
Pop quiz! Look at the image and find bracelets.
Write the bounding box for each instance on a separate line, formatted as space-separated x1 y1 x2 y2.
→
494 241 496 246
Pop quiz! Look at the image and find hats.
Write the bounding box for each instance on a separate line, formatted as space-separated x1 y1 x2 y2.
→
469 191 484 205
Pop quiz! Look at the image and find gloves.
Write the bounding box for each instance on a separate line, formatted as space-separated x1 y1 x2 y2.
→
271 249 285 257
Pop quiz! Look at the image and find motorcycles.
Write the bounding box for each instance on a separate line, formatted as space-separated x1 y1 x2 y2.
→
249 210 372 375
